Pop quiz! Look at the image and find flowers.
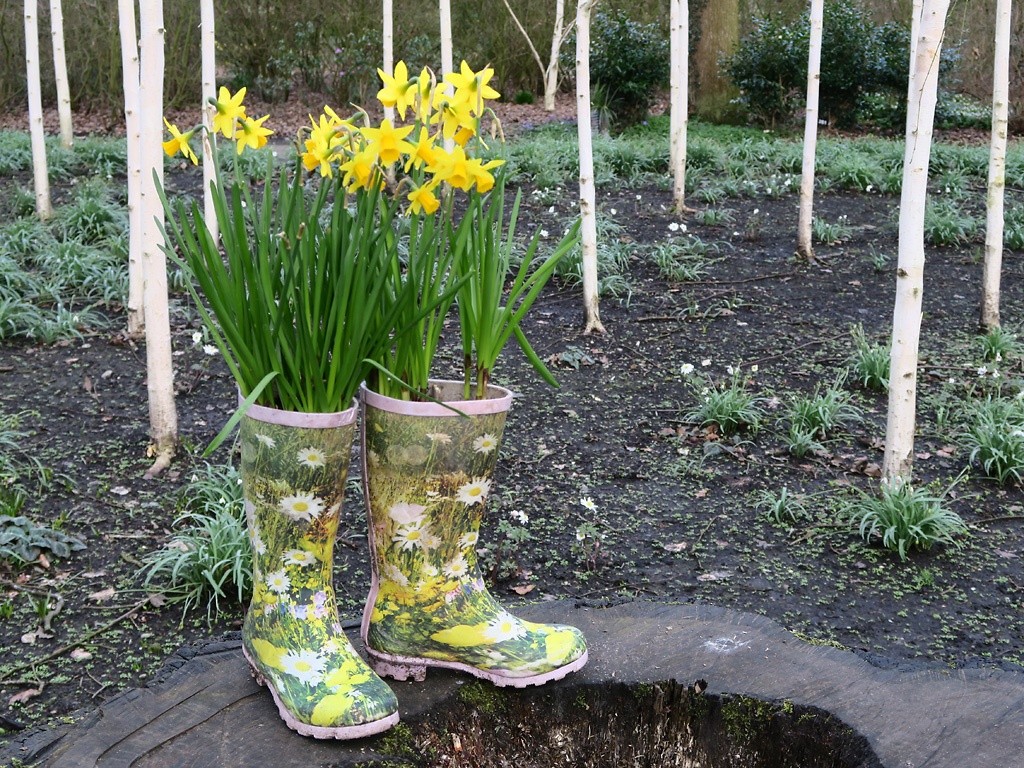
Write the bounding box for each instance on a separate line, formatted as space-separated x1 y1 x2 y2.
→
151 59 583 458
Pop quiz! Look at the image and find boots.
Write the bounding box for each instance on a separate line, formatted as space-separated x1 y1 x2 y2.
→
362 372 588 689
238 386 400 742
